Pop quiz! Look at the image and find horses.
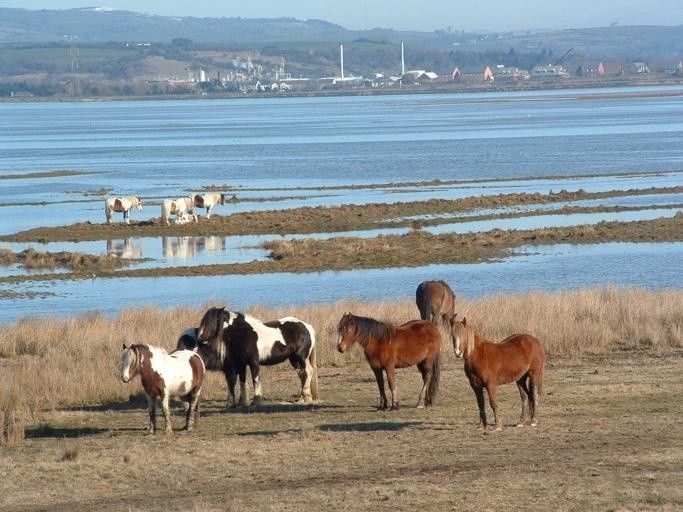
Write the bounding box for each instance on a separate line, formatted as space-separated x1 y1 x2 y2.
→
336 312 442 414
105 196 142 225
121 343 207 434
160 193 225 226
177 306 321 413
449 312 545 431
415 280 458 327
